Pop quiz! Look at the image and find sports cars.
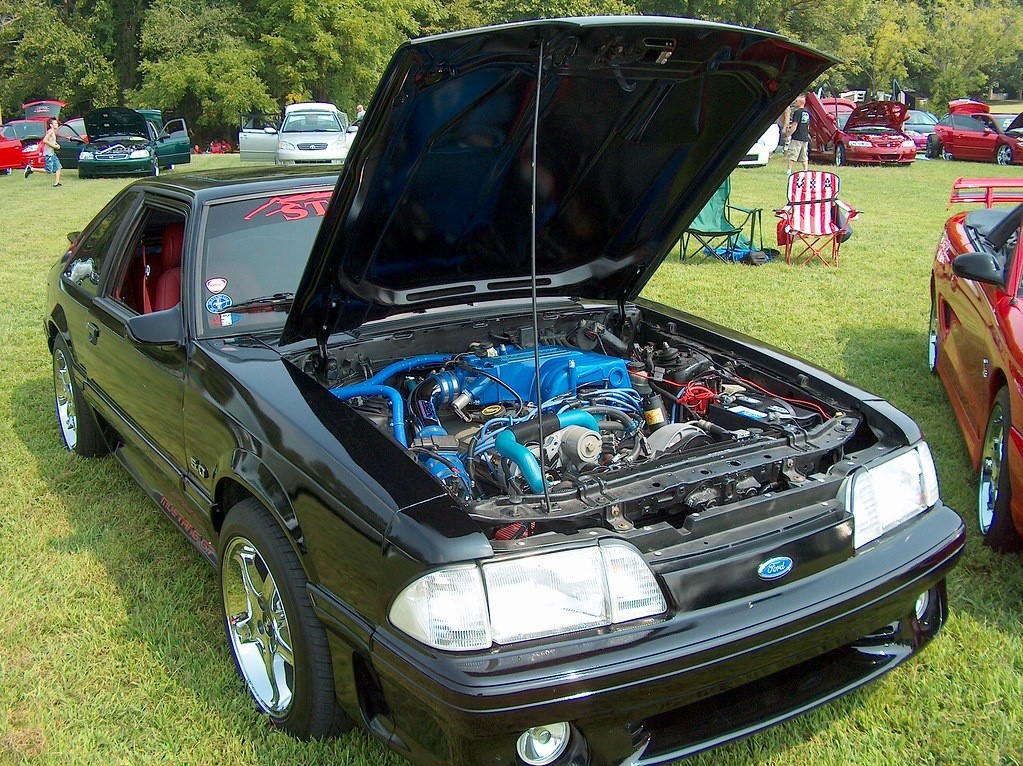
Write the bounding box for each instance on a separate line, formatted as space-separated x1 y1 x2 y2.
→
927 178 1023 548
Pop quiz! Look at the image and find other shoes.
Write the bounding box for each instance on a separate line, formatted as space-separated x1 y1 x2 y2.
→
24 165 33 178
53 183 62 187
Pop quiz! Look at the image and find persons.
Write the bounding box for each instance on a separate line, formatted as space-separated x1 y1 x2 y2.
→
25 116 64 187
356 105 366 125
208 139 233 154
193 145 203 154
777 105 791 154
188 128 195 145
786 97 810 175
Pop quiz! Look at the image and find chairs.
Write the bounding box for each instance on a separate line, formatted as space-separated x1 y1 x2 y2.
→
155 224 272 313
680 176 763 266
772 171 864 268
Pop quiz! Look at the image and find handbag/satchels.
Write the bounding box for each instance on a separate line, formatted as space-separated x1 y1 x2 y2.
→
742 251 767 265
831 205 853 242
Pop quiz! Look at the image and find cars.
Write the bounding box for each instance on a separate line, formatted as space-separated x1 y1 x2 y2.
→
0 101 88 172
905 109 939 150
238 100 367 167
57 106 191 178
739 124 781 166
805 76 916 166
926 99 1023 166
42 14 969 765
